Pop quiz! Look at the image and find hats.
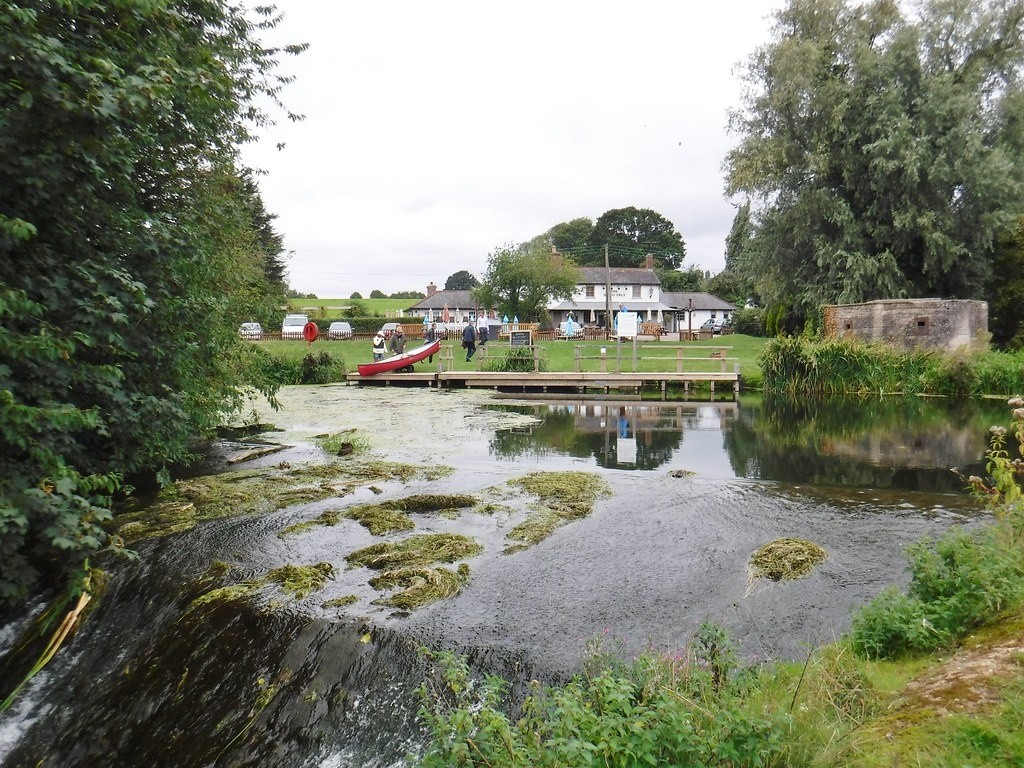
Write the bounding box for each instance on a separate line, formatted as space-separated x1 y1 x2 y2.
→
377 331 383 335
396 328 404 333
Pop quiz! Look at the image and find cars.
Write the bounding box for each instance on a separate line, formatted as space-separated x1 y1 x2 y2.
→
326 322 356 340
421 324 448 339
377 323 402 338
554 321 585 340
238 323 262 340
720 318 737 336
700 318 726 333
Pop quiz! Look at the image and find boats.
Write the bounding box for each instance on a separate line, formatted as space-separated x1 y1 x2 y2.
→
358 337 441 377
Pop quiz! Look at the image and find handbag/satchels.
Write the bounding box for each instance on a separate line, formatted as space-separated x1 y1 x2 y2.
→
462 339 467 349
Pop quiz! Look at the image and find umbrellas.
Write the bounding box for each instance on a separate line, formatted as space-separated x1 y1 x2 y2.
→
590 308 595 322
455 307 460 321
657 304 663 324
566 316 574 337
443 303 449 322
647 307 652 322
429 308 433 321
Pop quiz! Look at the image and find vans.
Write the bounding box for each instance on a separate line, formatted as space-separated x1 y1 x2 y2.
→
281 314 309 340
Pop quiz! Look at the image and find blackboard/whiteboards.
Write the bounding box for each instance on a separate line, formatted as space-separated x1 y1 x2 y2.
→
511 332 530 345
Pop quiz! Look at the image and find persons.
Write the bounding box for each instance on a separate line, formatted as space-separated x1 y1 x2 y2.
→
372 330 388 362
421 323 436 363
463 321 476 362
616 303 627 343
389 326 406 373
476 311 489 345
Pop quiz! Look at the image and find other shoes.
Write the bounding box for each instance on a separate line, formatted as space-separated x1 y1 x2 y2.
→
465 357 472 363
419 359 424 364
